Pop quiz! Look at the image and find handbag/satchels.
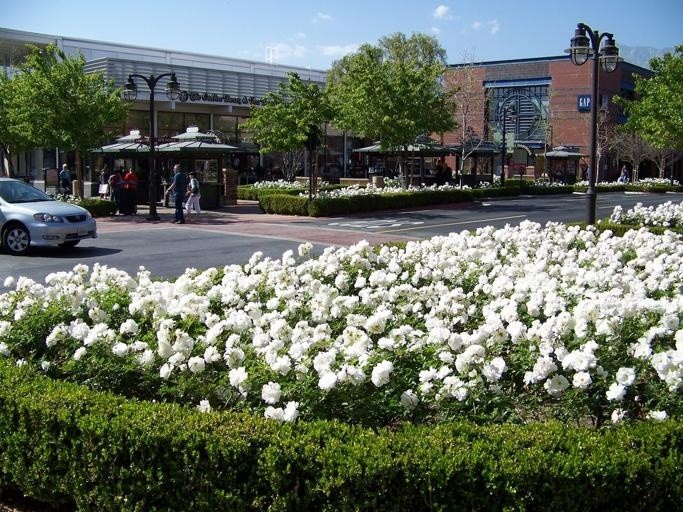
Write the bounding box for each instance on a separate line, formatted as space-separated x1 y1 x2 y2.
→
191 180 198 194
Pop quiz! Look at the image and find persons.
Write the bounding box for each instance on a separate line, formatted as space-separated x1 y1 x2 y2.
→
548 158 629 183
58 161 202 224
375 158 454 185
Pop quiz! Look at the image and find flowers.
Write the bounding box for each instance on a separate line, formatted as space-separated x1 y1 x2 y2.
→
252 178 493 200
535 177 681 187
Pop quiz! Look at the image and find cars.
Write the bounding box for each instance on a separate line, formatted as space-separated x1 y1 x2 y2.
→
0 176 98 255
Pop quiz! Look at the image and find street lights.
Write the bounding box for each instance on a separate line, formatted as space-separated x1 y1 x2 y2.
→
498 100 517 187
122 70 182 222
564 22 624 225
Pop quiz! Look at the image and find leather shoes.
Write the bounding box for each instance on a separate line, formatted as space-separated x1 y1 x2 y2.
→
169 219 178 223
174 220 185 224
